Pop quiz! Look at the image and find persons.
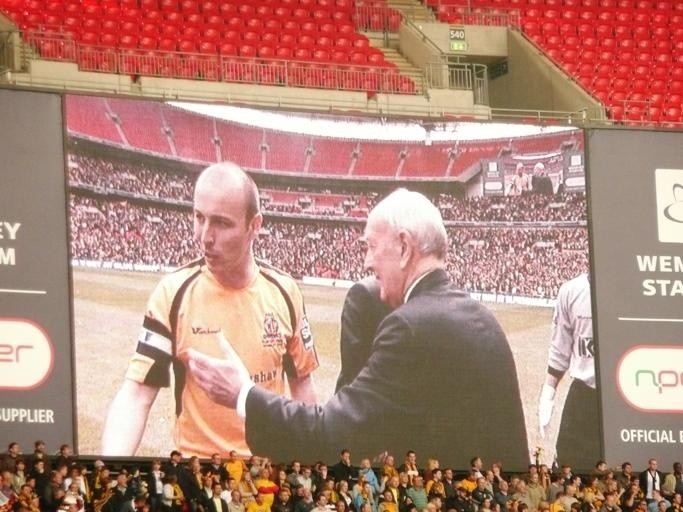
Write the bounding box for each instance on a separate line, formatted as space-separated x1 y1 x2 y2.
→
0 439 681 510
61 94 602 474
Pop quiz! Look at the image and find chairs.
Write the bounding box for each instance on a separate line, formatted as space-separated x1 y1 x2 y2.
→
0 0 683 129
